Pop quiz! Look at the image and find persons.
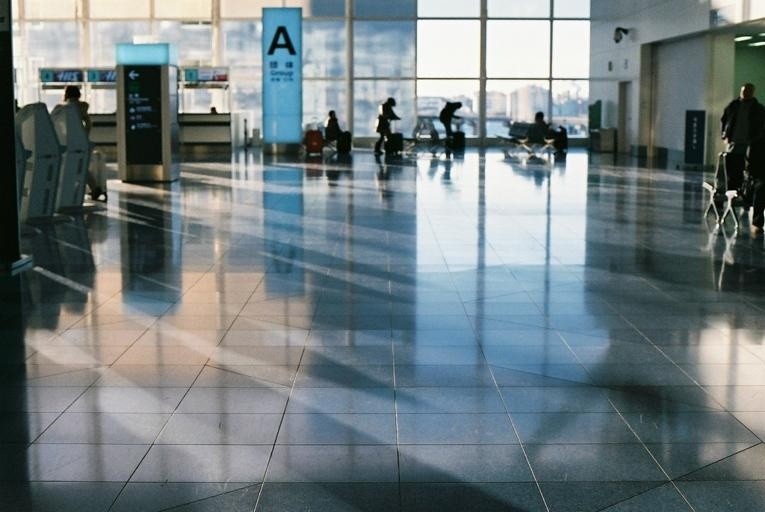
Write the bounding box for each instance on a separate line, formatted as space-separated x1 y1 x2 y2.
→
210 106 218 114
439 101 463 137
326 110 353 154
721 83 762 191
62 83 99 196
373 97 401 154
534 105 570 157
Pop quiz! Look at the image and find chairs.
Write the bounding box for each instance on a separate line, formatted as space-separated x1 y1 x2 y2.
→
703 147 751 230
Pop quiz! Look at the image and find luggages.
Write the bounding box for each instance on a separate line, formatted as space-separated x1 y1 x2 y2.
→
392 133 404 152
306 130 323 153
453 131 465 153
344 131 352 152
85 150 107 194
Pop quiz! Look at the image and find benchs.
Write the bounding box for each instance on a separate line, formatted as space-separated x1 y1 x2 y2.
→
497 122 565 164
